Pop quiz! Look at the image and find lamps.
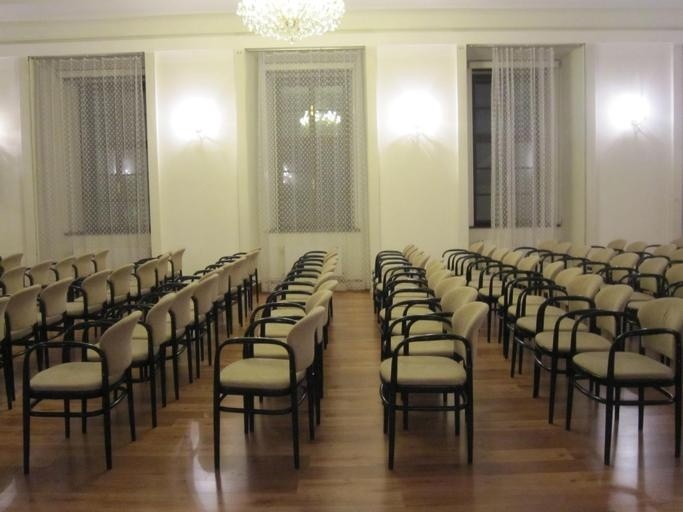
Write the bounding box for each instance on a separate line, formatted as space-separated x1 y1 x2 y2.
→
236 0 345 46
